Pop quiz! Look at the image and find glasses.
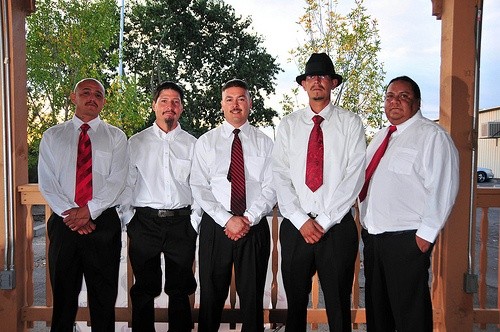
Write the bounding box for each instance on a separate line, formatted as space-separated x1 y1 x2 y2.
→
306 75 325 80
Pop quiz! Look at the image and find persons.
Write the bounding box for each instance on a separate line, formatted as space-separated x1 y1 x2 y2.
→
38 78 127 332
357 75 460 332
118 82 205 332
270 52 367 332
189 79 278 332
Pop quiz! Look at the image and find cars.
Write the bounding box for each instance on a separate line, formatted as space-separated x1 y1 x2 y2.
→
477 167 494 183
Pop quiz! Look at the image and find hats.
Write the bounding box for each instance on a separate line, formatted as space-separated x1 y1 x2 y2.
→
296 53 342 87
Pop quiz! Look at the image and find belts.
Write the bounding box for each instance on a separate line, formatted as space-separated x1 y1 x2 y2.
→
307 212 318 217
136 208 190 216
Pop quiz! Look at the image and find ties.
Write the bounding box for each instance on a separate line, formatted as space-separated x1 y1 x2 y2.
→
306 115 324 192
74 123 92 208
227 129 246 217
359 125 397 203
